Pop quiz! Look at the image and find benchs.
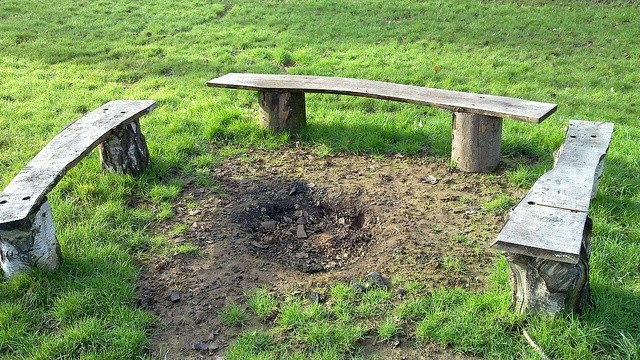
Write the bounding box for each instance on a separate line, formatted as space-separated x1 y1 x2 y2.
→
490 120 615 323
1 99 159 279
206 72 558 172
